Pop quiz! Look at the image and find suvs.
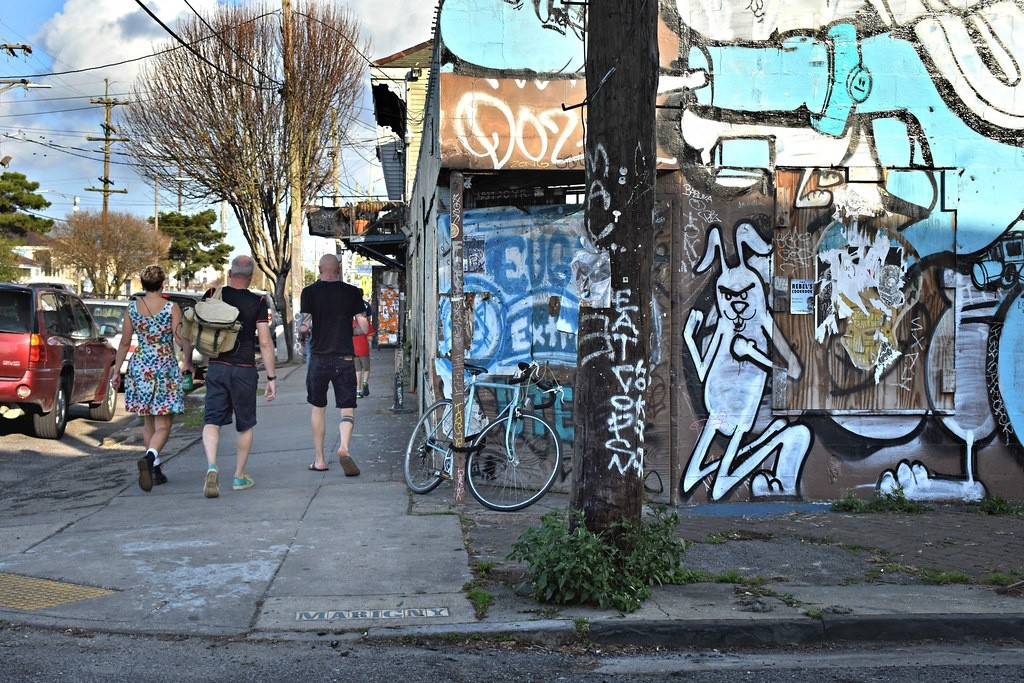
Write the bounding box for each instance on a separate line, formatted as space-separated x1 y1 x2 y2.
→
0 284 118 439
21 276 277 392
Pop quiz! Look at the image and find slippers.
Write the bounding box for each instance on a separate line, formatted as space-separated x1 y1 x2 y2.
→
339 456 360 476
307 461 329 471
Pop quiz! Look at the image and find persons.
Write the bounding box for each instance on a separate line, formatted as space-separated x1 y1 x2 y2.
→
111 266 182 491
297 253 369 479
358 288 372 321
179 254 276 498
353 313 376 399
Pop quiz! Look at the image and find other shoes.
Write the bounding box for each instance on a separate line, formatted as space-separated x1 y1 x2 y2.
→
356 392 363 397
361 382 369 396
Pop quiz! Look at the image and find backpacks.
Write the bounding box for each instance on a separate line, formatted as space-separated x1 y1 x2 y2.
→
178 286 242 358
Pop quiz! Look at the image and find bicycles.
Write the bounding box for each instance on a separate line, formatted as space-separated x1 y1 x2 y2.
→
404 363 566 511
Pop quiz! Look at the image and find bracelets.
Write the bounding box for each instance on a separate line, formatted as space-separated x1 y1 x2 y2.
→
267 375 276 381
297 337 305 342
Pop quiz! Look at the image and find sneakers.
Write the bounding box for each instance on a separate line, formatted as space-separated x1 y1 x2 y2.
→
151 463 168 485
232 473 255 490
136 455 154 491
204 465 221 498
